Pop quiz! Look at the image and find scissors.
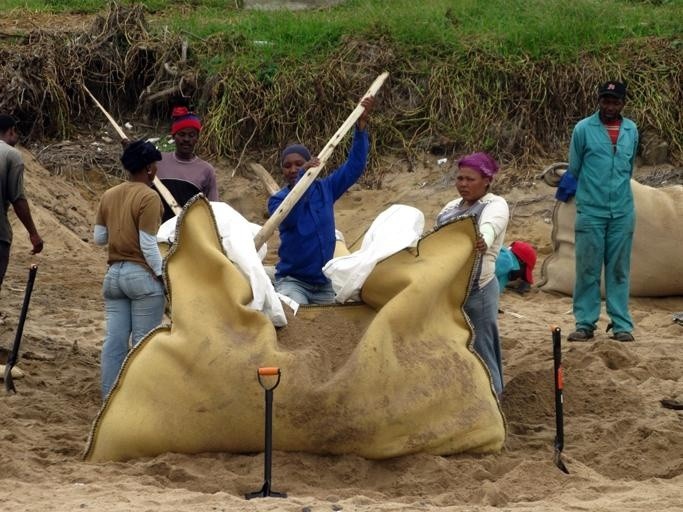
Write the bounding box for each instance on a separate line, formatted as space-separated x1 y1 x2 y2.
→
5 265 37 395
549 324 571 475
245 367 288 501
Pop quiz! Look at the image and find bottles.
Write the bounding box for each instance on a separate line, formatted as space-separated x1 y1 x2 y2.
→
510 240 536 284
121 139 162 169
598 81 625 100
458 152 499 177
171 106 201 137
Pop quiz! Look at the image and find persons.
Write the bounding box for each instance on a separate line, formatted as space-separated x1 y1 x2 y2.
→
0 112 43 294
92 141 170 403
267 95 376 308
434 153 510 399
120 106 219 256
567 80 639 343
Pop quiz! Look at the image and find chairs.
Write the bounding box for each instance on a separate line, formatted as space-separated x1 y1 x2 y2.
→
613 331 633 342
568 328 593 341
